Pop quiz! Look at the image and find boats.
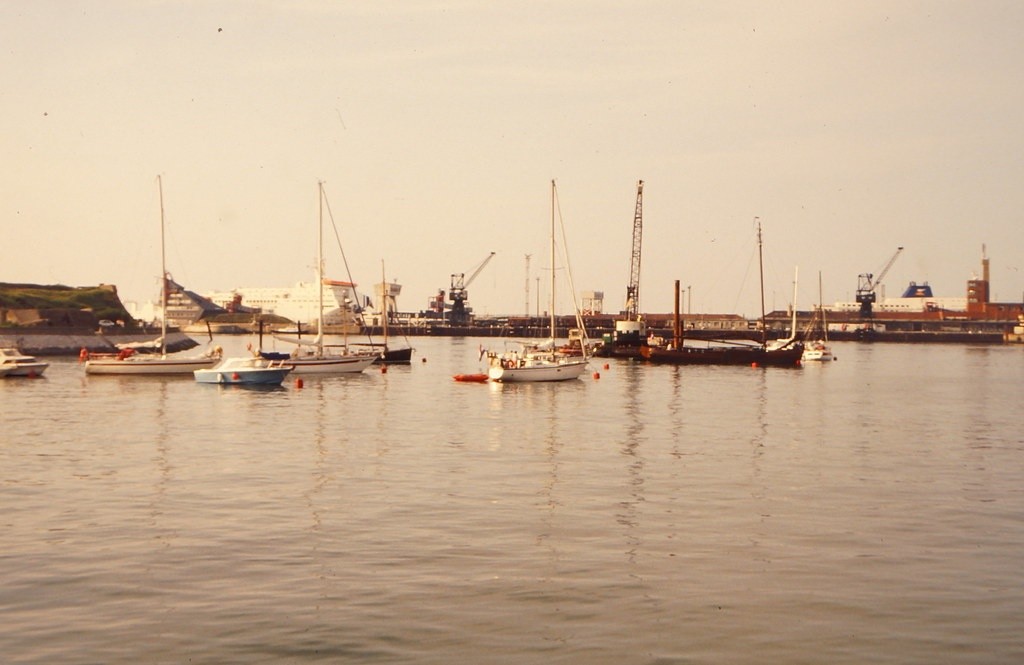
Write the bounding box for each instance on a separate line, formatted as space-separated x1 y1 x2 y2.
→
1002 303 1024 346
193 357 295 386
812 281 967 311
0 346 49 377
268 323 309 334
599 281 804 369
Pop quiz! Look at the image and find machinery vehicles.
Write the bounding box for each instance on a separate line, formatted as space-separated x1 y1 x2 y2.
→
614 179 648 337
418 251 499 325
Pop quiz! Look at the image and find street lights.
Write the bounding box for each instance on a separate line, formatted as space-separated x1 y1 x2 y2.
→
681 290 685 314
688 285 691 313
536 276 541 317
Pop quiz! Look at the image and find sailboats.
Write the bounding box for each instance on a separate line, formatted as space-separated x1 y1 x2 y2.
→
806 271 839 363
85 176 224 376
720 215 801 350
486 178 595 381
248 179 382 377
321 259 413 365
